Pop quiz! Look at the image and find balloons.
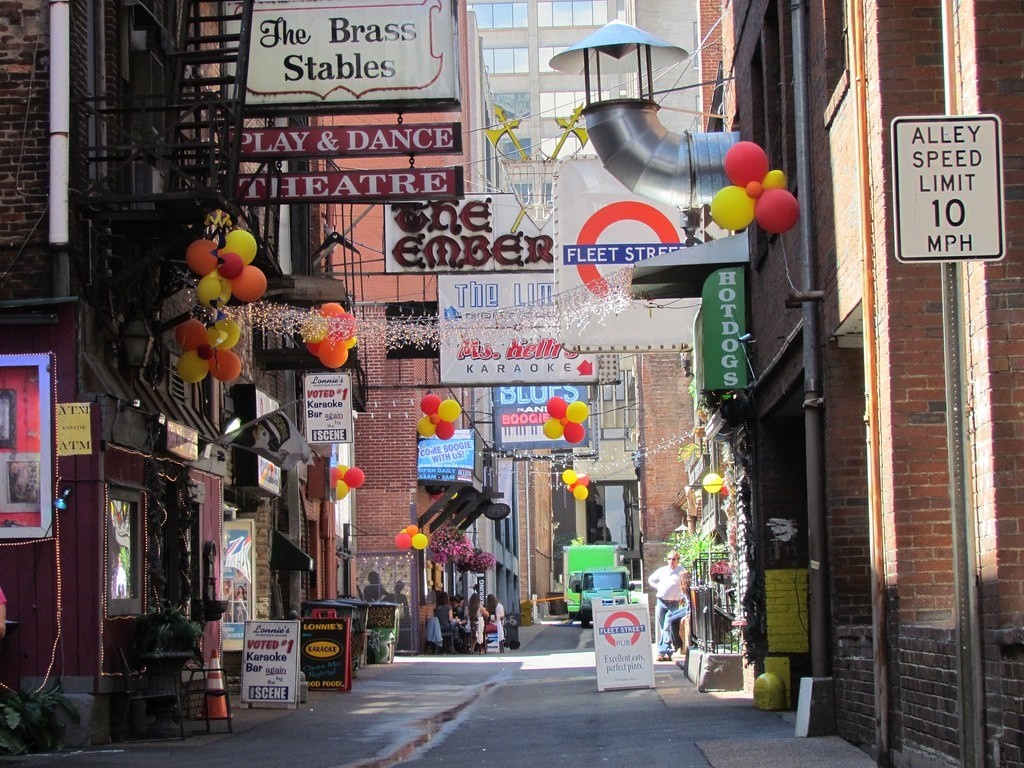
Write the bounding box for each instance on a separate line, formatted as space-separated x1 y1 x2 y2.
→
702 473 730 495
395 525 428 550
300 303 357 369
543 397 589 443
176 318 242 382
562 470 589 500
187 229 266 308
416 394 461 439
335 464 365 501
710 141 799 233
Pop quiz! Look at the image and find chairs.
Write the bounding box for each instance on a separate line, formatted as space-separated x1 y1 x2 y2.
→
118 646 187 744
180 645 234 735
426 617 454 655
483 631 505 654
458 621 486 654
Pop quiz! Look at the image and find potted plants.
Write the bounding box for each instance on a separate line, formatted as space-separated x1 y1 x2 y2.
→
130 600 206 654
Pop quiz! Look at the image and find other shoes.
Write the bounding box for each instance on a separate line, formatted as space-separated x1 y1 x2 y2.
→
658 654 671 661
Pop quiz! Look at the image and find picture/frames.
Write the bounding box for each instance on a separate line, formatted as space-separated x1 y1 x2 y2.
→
105 486 142 617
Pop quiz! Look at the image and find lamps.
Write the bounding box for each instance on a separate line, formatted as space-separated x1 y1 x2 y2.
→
222 417 242 435
336 547 354 561
53 485 73 511
679 343 697 378
738 333 758 344
674 516 689 531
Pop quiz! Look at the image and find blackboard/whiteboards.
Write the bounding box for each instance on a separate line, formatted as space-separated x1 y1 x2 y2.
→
298 617 353 688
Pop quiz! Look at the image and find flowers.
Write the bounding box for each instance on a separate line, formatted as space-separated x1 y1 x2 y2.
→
431 522 497 573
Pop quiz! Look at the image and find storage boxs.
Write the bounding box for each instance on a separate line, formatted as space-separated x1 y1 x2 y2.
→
486 636 499 652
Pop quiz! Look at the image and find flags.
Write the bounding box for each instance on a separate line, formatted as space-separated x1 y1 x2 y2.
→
221 410 313 469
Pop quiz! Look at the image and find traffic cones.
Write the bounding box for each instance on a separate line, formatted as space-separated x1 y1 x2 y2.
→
193 648 234 721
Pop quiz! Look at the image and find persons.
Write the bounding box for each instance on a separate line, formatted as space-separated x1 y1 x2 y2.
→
647 551 687 661
435 592 505 653
665 569 695 653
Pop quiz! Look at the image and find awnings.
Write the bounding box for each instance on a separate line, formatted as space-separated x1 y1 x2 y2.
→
271 528 314 571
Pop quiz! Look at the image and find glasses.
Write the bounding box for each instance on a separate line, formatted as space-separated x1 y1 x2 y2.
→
668 557 678 562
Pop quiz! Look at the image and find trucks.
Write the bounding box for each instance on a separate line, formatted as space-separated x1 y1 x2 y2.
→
563 543 642 629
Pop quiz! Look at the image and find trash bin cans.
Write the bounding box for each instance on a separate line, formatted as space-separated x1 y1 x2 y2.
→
505 613 526 649
